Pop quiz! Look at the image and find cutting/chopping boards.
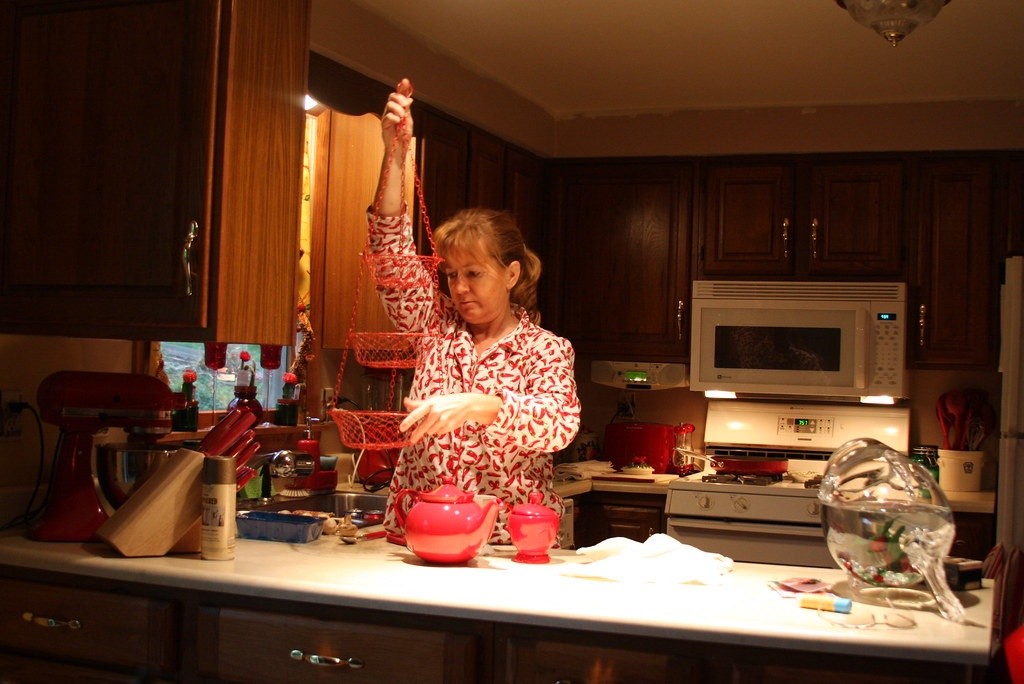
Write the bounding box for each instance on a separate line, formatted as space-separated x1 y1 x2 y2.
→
592 474 679 484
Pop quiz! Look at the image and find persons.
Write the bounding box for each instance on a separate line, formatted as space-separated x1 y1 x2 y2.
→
367 91 582 552
719 321 830 389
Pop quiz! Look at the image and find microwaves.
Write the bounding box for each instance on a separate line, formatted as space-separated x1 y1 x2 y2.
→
690 279 916 405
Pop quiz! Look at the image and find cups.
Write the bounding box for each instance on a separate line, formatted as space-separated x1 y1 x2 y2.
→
571 433 600 462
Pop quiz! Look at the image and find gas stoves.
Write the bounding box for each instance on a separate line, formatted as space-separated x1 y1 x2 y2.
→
665 401 910 523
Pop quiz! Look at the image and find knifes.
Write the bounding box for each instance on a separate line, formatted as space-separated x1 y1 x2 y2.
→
194 407 260 470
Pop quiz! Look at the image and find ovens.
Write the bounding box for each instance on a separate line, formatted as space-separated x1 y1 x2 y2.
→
667 518 841 569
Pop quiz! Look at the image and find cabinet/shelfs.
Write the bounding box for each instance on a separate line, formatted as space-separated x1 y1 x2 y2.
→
0 0 311 346
951 514 998 560
0 562 970 684
312 113 1023 368
571 491 667 547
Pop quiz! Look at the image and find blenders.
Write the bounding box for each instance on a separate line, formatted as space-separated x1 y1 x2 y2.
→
28 371 179 542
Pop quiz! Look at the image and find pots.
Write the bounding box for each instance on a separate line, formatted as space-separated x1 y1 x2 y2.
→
605 423 675 474
677 447 788 475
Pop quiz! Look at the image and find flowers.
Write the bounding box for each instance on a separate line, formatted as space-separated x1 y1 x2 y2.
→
235 351 257 386
280 373 297 399
181 369 197 403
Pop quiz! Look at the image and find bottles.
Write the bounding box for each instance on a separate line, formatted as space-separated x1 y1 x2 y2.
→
800 593 852 614
673 423 695 476
201 457 237 560
911 446 939 484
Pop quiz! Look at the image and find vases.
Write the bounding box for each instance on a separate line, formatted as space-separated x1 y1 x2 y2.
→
275 400 300 425
171 401 200 433
227 387 263 428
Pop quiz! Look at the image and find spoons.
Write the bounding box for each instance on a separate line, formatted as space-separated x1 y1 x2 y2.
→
340 531 387 544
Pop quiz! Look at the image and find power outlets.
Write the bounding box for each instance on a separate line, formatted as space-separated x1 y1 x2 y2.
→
1 390 24 442
617 392 635 419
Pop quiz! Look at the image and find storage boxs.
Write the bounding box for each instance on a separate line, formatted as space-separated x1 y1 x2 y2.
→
237 510 329 546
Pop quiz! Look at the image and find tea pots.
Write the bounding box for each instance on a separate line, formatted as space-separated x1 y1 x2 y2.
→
394 476 504 565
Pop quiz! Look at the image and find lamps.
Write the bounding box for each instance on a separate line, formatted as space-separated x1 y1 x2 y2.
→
835 0 952 47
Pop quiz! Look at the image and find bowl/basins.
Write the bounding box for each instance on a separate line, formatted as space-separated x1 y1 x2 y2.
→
321 457 337 471
505 492 559 564
236 512 328 543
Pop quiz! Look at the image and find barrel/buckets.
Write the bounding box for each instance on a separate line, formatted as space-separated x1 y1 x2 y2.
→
937 448 984 492
